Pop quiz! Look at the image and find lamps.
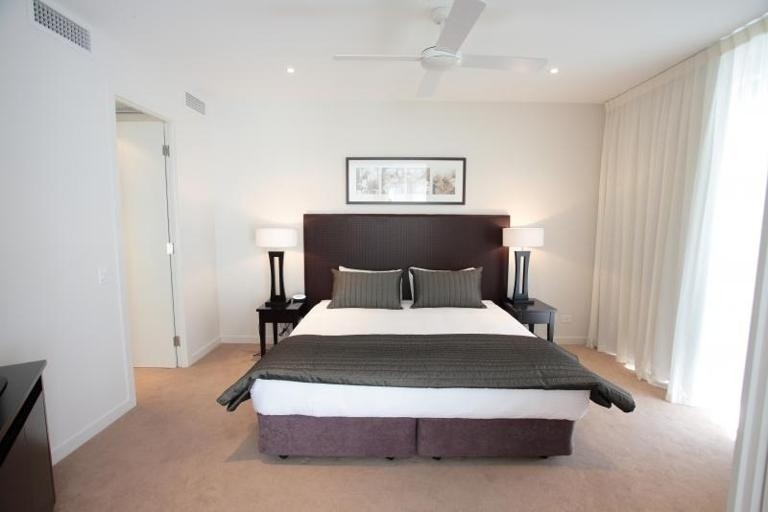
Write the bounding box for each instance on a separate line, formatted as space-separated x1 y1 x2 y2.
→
503 228 545 304
257 226 298 304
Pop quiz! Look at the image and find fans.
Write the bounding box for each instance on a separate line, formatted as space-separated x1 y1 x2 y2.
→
334 0 547 100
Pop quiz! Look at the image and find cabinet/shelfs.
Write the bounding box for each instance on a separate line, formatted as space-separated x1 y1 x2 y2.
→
1 359 57 512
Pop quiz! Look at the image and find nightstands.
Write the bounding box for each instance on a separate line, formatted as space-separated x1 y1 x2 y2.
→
255 297 303 358
503 296 558 341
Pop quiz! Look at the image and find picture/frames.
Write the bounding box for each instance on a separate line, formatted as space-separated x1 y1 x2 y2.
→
344 156 467 206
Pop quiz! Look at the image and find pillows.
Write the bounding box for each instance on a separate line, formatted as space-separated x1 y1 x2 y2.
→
327 267 404 310
338 265 403 303
407 267 477 304
410 265 491 308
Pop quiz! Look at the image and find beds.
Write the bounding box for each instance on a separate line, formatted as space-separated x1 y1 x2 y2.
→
216 213 636 462
